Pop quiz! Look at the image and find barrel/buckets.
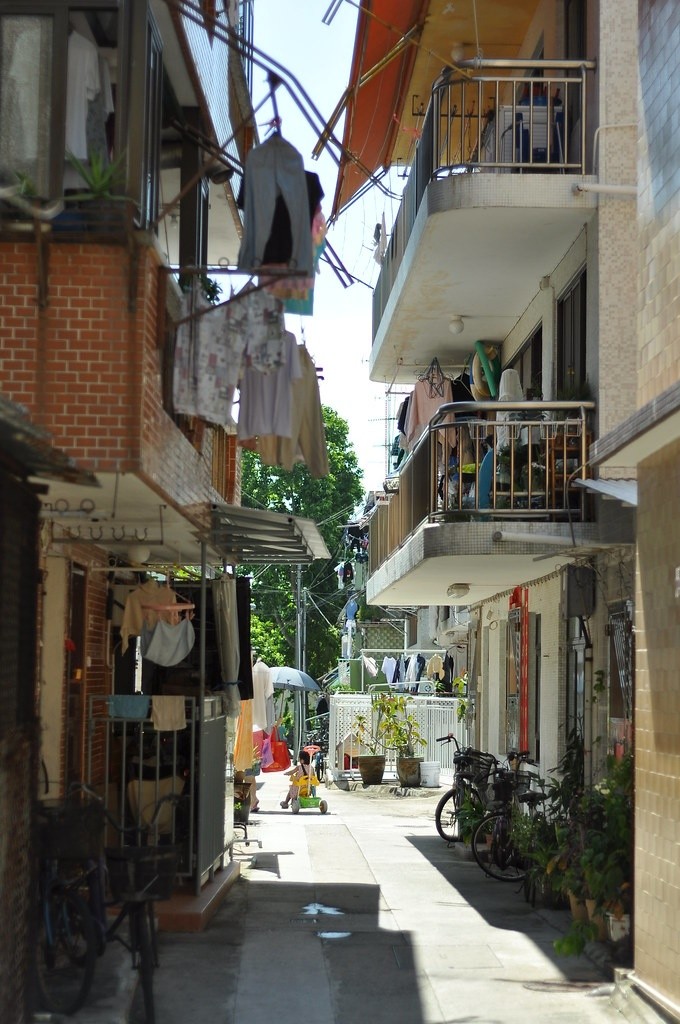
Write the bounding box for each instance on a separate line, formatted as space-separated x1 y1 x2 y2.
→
419 761 441 788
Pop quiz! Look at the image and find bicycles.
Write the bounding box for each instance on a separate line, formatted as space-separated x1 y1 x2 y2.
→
302 730 328 781
468 748 555 909
433 734 497 846
31 783 202 1024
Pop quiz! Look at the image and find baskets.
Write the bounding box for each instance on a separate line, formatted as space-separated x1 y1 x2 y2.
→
454 746 493 784
504 769 531 793
103 846 177 903
31 801 104 862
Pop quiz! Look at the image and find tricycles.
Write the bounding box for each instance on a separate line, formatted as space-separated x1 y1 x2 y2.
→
284 745 328 814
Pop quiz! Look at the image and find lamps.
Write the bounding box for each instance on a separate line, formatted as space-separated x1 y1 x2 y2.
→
448 315 464 336
447 585 470 598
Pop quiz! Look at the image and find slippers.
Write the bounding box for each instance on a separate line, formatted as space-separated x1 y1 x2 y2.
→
250 806 259 813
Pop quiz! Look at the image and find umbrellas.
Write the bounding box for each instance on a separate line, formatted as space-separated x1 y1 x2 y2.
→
270 666 322 718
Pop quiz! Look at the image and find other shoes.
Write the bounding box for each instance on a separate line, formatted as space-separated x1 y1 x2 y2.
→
281 801 288 808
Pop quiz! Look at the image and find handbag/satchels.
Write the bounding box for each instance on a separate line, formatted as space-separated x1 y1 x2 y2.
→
261 726 290 772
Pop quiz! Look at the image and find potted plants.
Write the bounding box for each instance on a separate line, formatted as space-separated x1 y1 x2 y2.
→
234 745 262 823
67 148 145 233
449 713 632 957
354 688 427 787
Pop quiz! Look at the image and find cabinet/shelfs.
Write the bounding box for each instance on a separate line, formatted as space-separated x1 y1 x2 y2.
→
480 105 562 173
541 431 595 521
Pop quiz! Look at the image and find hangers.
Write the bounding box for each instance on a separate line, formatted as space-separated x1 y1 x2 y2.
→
416 352 473 399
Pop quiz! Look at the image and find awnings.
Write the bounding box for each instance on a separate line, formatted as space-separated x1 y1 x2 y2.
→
209 504 331 561
329 0 431 222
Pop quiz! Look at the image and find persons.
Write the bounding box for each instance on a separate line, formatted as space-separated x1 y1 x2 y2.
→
278 726 293 758
280 751 314 808
245 730 269 813
